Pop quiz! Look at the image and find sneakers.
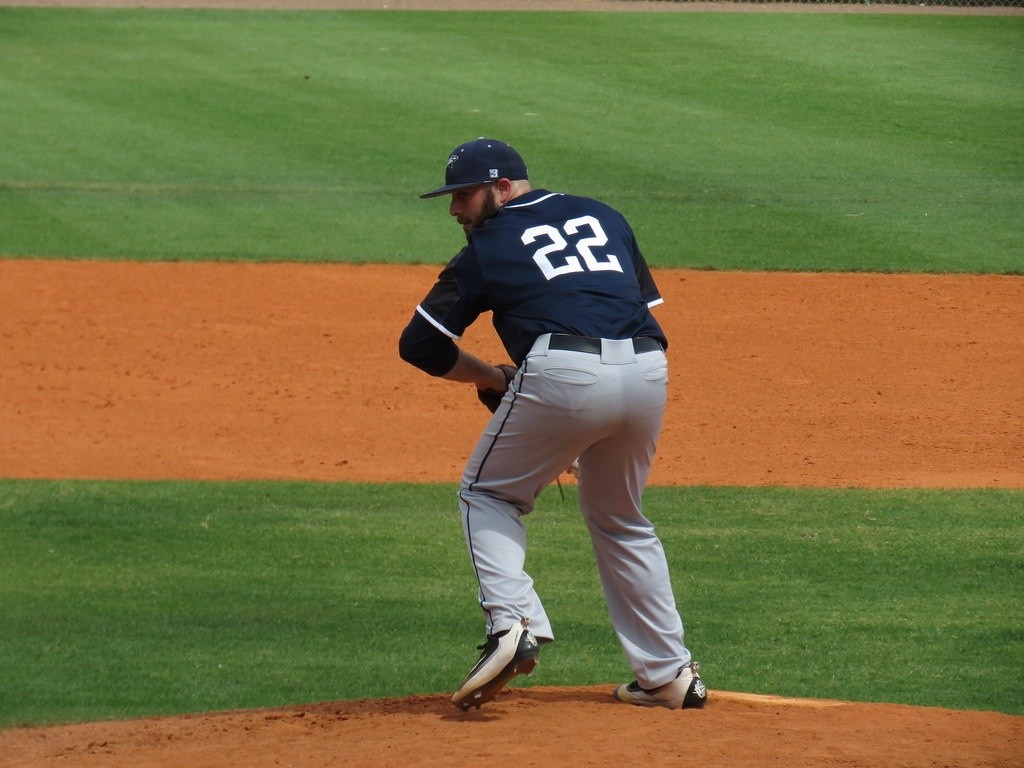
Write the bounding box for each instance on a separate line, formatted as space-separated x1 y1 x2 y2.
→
450 617 539 711
615 662 706 710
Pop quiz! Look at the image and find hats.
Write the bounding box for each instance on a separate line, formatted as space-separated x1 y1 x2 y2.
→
420 137 528 199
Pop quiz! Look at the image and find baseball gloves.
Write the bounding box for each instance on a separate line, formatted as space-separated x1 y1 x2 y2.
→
475 364 519 416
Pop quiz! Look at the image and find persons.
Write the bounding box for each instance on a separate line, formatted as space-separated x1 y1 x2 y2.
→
399 135 707 711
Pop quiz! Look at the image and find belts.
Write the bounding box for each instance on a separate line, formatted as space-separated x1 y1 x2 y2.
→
547 334 663 354
